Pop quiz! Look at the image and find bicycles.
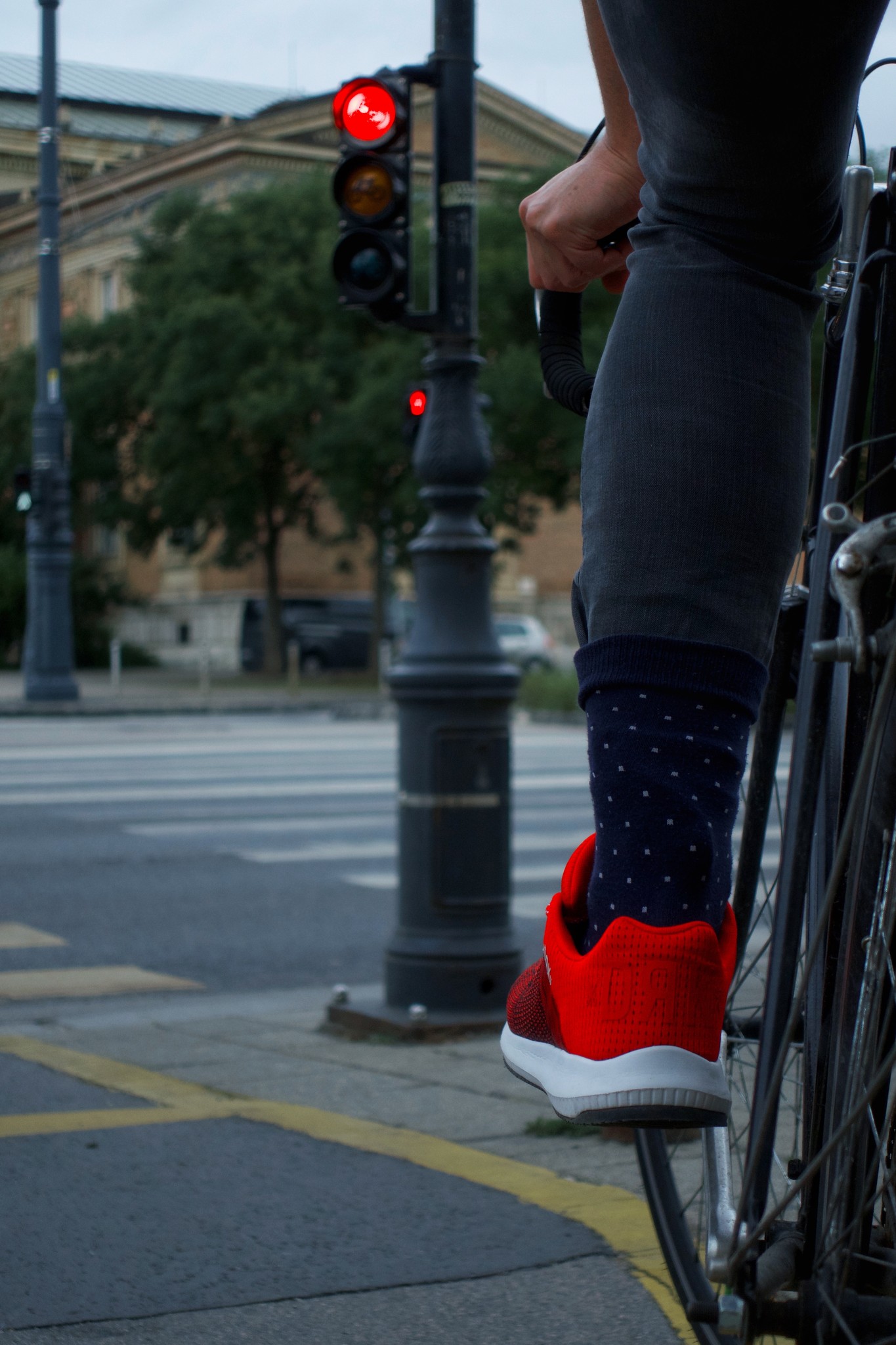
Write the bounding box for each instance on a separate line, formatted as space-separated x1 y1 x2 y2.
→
526 56 896 1339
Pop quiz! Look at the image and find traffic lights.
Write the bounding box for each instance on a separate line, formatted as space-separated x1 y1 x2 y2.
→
326 78 413 325
410 391 425 434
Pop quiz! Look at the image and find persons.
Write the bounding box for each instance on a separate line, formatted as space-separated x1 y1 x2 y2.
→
500 1 895 1127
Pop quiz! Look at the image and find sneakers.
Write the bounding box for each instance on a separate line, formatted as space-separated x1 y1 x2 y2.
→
500 831 734 1130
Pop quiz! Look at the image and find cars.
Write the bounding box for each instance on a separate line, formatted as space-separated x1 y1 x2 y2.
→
282 597 413 677
495 618 562 679
